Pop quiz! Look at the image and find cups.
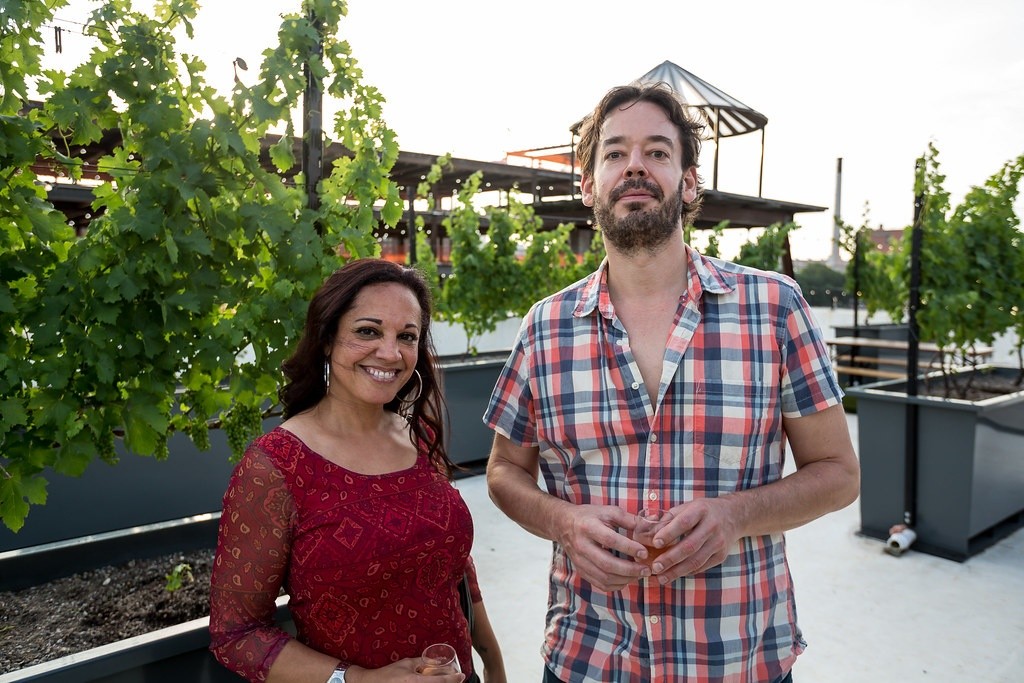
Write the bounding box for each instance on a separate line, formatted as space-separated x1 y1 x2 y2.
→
632 508 681 570
414 643 462 677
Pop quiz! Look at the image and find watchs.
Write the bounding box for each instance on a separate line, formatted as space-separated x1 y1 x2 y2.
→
326 660 352 683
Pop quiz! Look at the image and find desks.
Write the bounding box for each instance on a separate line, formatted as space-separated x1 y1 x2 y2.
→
824 338 992 377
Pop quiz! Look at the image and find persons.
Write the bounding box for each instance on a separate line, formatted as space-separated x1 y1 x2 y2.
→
482 80 861 683
208 257 509 683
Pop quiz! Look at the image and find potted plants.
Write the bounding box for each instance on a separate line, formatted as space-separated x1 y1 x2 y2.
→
828 210 938 385
842 137 1024 565
418 152 582 474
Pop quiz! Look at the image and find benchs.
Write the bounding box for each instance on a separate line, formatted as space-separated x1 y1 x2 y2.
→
833 354 953 383
